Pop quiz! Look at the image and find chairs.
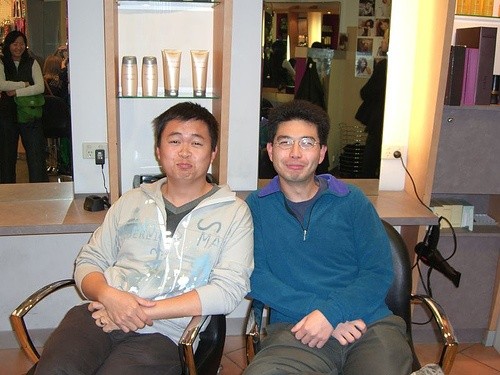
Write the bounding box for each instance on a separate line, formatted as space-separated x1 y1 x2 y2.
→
9 280 226 375
243 219 458 375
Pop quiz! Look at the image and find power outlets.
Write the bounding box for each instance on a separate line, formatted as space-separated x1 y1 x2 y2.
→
83 143 108 159
381 146 403 160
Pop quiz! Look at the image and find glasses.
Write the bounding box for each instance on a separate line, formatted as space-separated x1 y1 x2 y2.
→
272 137 321 150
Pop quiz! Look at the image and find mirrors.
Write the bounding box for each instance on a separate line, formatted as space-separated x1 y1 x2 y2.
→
257 1 394 179
0 1 76 184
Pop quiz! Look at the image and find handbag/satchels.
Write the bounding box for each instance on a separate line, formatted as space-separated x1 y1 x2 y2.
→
14 94 45 123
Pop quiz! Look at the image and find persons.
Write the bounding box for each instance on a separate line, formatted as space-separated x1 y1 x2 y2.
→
355 0 391 78
260 38 336 109
242 100 414 374
25 102 255 375
355 29 389 179
0 31 72 184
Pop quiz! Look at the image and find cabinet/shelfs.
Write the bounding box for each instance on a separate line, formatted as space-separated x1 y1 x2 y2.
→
402 0 500 348
103 0 233 206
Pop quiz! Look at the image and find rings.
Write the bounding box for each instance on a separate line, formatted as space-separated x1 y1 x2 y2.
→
98 318 104 326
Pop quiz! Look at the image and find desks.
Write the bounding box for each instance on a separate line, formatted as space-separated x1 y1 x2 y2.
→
0 191 438 236
